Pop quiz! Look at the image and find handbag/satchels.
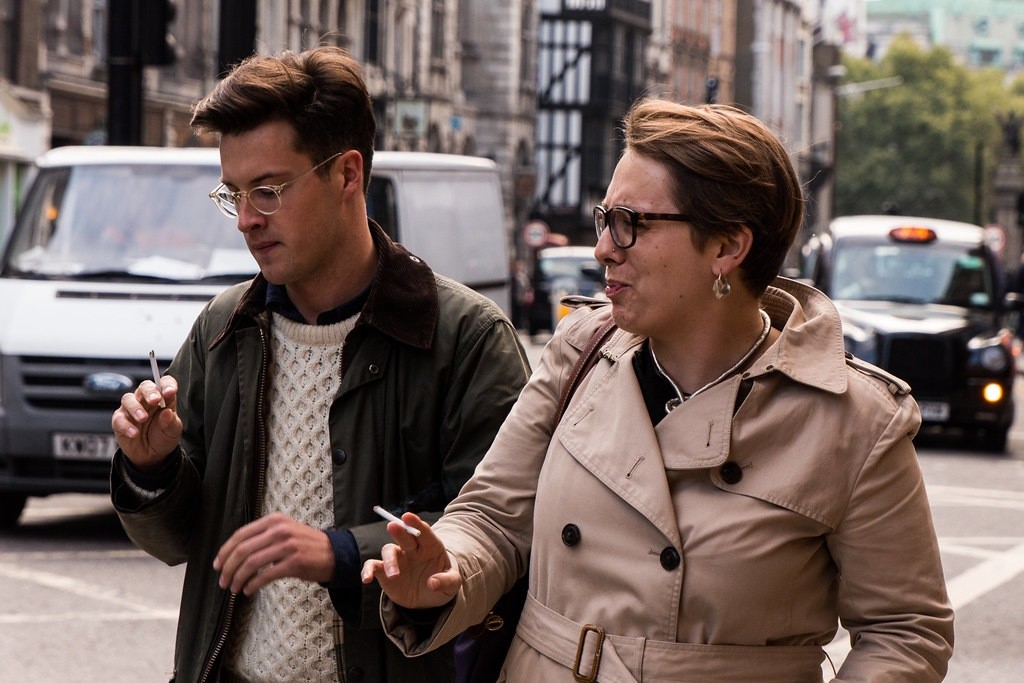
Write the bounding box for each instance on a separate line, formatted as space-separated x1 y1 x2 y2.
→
453 313 618 683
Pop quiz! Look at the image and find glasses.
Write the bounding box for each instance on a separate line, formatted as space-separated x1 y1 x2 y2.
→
592 205 693 249
208 153 343 219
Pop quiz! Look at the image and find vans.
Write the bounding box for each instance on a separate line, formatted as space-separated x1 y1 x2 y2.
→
0 146 512 522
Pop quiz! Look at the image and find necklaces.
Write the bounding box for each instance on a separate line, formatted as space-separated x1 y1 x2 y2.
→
648 309 771 414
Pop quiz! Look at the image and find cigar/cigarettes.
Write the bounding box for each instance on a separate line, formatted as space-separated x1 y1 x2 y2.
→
374 506 420 536
149 350 165 408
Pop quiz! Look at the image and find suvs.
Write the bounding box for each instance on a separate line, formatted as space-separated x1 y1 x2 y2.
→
804 213 1024 452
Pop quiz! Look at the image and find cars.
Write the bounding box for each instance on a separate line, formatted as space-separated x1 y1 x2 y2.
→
526 245 609 339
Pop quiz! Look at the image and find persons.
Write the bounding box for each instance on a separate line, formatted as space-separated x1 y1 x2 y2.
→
109 48 532 683
361 98 956 683
